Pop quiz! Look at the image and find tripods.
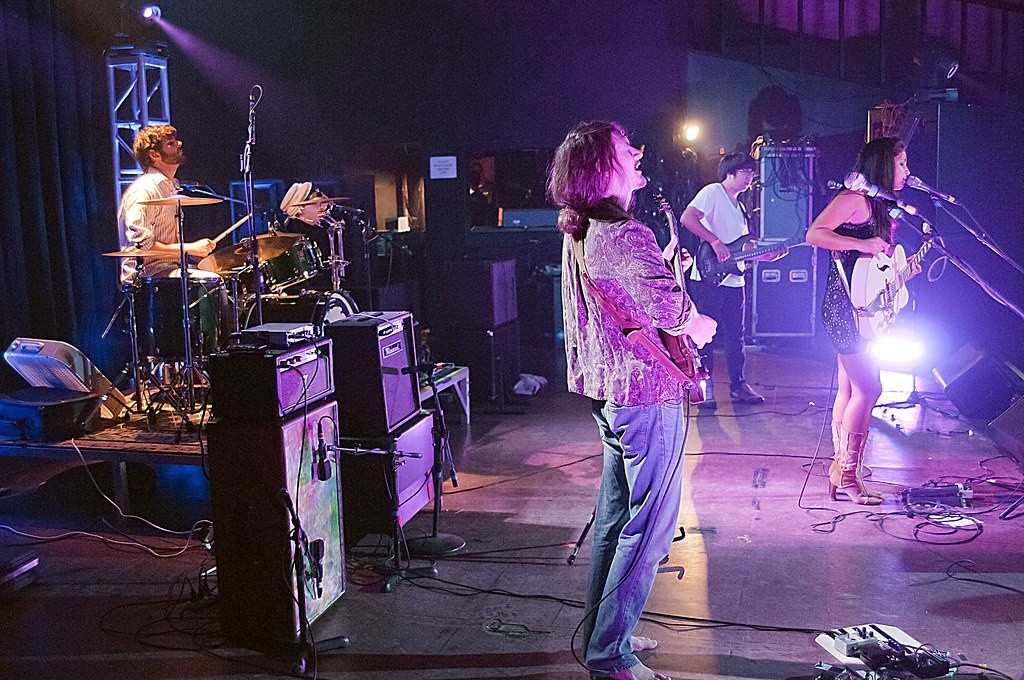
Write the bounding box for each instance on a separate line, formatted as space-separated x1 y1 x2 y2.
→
872 257 960 419
81 294 197 434
144 210 210 442
373 458 438 593
234 543 350 676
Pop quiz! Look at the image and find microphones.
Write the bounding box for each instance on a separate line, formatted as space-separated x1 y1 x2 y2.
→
827 169 916 220
315 422 332 483
401 363 454 375
307 539 326 600
906 175 956 204
326 202 365 214
248 94 257 146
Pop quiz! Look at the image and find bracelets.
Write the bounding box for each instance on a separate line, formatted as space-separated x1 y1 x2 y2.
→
710 239 720 246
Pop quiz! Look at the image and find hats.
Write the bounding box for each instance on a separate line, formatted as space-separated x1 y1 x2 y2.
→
280 181 313 218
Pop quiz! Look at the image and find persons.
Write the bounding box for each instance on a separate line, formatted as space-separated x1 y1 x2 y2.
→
680 151 788 407
118 125 219 358
282 183 340 263
547 121 718 680
467 162 503 213
805 136 922 504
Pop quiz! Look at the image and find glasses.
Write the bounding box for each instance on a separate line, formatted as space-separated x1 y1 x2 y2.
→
735 168 756 177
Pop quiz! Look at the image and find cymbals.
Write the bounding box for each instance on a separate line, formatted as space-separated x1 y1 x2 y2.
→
240 231 307 243
101 249 178 256
291 196 352 207
197 236 298 274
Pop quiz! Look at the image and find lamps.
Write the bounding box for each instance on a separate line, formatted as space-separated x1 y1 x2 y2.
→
120 0 161 39
924 40 959 80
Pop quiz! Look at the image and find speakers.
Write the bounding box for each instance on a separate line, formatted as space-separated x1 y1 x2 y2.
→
987 395 1024 464
207 401 346 641
932 342 1016 417
211 338 335 417
324 310 440 530
434 258 521 401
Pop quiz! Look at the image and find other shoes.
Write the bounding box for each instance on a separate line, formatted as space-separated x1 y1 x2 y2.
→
730 382 766 405
697 380 717 408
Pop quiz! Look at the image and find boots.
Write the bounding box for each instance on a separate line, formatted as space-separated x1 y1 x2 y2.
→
829 423 884 505
828 421 884 496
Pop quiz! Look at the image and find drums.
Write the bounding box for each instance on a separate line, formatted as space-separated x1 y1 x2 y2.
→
257 235 321 294
128 276 224 364
228 289 361 341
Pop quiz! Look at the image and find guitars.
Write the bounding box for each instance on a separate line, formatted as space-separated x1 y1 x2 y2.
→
849 239 932 342
651 192 712 405
696 234 806 289
